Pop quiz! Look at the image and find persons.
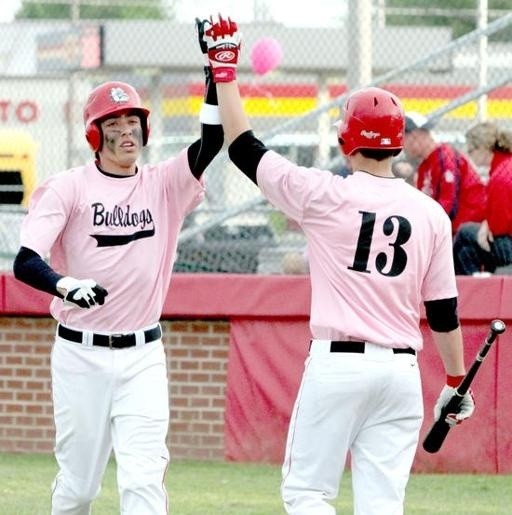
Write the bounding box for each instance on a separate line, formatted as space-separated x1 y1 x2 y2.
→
400 110 487 238
450 119 511 273
204 13 474 515
14 17 225 515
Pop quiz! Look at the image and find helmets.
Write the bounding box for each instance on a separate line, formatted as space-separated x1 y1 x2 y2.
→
334 86 407 157
82 78 154 154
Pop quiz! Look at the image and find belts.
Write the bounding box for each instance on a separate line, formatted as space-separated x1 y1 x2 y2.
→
307 339 418 360
53 323 163 352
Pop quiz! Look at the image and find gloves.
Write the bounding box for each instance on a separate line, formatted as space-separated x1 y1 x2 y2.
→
53 275 109 310
199 8 243 88
432 373 477 429
193 12 223 82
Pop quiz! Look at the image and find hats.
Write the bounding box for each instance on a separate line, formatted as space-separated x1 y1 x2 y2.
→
403 111 433 132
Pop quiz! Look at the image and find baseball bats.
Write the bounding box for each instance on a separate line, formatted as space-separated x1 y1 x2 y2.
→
423 319 505 453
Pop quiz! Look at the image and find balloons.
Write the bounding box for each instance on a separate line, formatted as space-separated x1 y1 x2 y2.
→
249 34 285 76
